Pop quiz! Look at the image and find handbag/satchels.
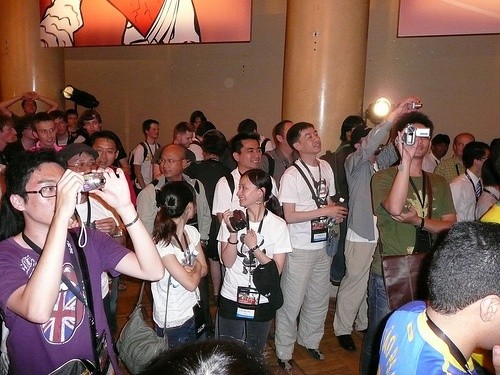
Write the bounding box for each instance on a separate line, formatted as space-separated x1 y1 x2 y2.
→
48 358 103 375
381 251 436 311
116 304 168 375
327 225 340 257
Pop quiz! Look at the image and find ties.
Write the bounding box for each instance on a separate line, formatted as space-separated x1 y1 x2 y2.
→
476 181 482 197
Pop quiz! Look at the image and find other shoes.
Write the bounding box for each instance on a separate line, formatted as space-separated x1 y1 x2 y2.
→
308 348 324 360
279 358 292 370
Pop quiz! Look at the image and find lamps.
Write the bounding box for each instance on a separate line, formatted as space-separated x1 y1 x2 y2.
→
61 85 99 112
366 97 395 127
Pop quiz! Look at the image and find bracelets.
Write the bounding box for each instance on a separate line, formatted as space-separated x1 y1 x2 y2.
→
125 212 139 227
415 218 424 230
251 245 258 252
228 238 238 244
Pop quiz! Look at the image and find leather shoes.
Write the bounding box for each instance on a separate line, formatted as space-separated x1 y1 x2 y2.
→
338 335 356 351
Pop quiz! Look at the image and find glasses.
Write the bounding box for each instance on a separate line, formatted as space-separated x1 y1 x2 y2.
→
67 162 100 171
16 186 58 197
157 159 185 165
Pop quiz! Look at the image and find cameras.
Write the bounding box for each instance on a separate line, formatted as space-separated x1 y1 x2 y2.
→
402 123 431 146
80 171 106 192
230 209 247 232
410 100 423 109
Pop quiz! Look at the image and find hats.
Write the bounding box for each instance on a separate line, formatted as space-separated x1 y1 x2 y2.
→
253 259 284 310
58 144 99 161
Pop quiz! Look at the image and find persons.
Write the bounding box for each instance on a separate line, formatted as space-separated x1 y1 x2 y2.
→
0 91 500 375
